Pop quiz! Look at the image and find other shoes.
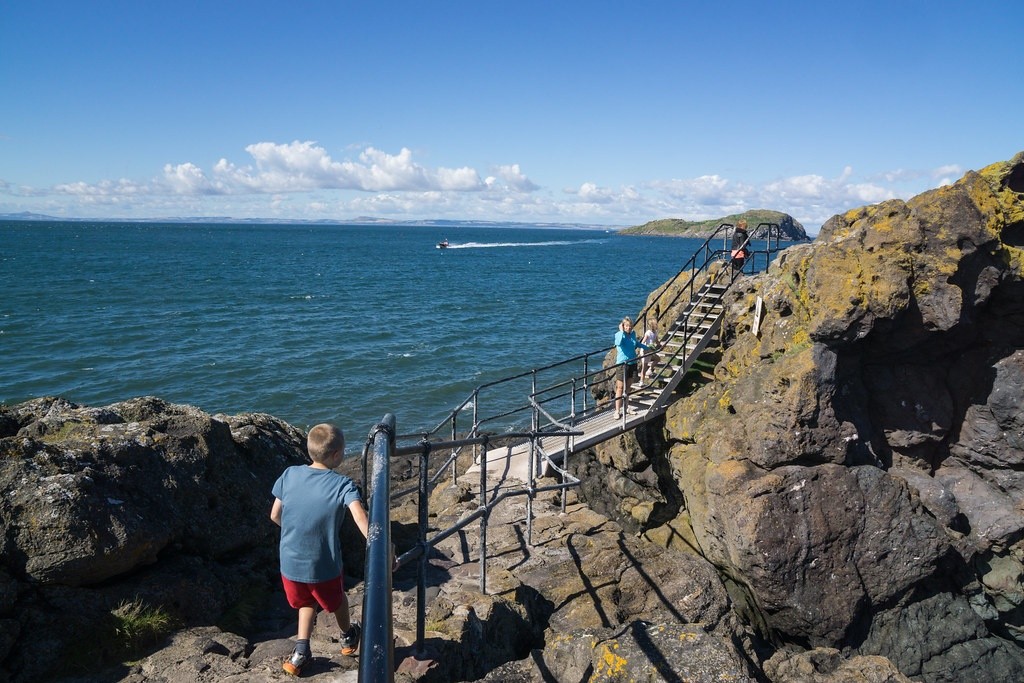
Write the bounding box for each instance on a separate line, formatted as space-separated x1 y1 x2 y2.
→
638 382 647 387
626 407 637 415
645 370 657 377
614 409 621 420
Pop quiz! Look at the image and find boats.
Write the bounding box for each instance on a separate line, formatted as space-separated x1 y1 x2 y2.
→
438 241 451 249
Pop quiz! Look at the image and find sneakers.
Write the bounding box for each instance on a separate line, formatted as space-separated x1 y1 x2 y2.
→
282 646 312 677
338 619 362 655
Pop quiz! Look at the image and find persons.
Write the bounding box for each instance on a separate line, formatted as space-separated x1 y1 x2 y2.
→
271 423 399 675
730 218 751 273
639 318 662 387
614 316 653 420
